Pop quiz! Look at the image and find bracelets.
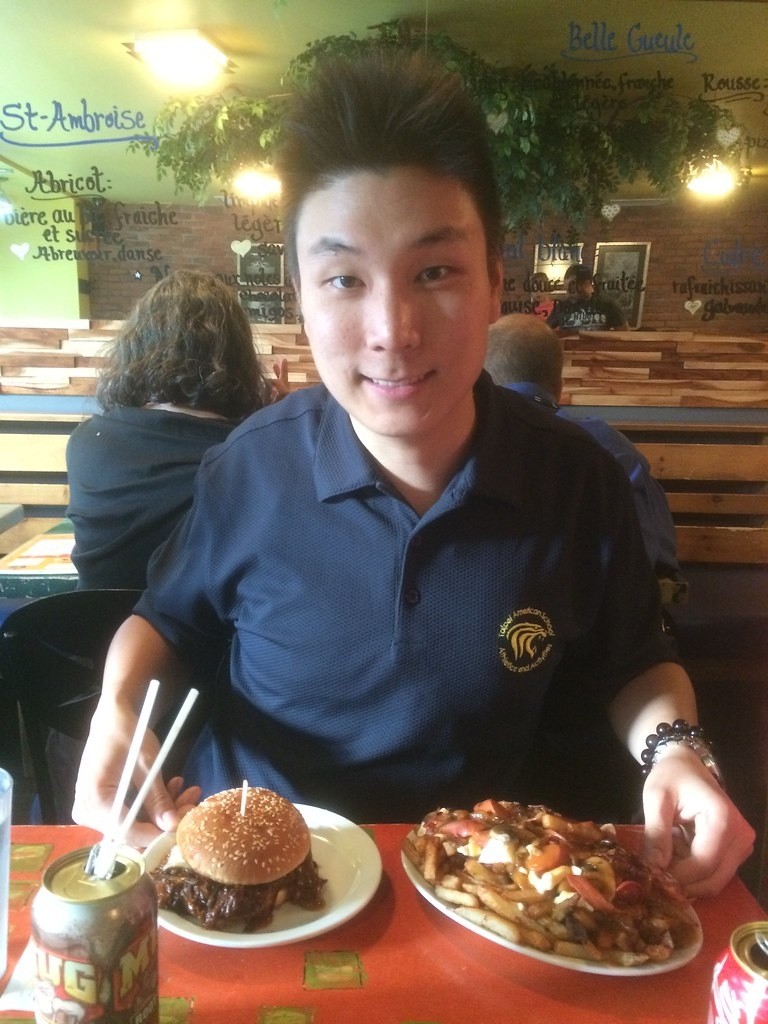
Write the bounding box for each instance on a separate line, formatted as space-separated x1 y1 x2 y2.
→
640 719 710 778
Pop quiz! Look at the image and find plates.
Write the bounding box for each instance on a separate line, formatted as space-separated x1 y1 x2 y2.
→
141 804 384 949
399 828 705 977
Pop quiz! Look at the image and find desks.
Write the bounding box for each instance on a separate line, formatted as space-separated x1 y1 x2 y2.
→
0 394 768 527
0 519 79 599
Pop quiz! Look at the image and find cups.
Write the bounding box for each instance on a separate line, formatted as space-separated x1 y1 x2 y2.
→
0 767 12 981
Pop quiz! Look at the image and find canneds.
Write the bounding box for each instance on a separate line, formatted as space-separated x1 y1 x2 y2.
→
707 920 768 1024
30 843 163 1024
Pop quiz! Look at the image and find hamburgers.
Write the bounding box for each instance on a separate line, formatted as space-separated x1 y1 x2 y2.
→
154 785 329 932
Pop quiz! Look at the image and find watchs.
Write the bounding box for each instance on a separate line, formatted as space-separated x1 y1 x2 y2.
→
651 733 724 791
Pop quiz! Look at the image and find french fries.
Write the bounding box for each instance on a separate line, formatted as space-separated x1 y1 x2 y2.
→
400 834 671 967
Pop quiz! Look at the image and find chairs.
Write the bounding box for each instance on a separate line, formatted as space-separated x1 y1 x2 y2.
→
0 586 142 825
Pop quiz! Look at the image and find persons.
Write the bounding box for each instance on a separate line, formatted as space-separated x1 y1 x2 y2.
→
483 313 681 581
529 272 556 320
73 33 756 901
65 272 290 591
545 265 630 332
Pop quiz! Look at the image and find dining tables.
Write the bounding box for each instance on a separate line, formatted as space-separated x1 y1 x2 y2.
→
0 824 768 1024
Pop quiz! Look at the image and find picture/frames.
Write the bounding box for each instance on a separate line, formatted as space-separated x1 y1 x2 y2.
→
592 241 652 330
237 242 285 287
237 290 285 324
